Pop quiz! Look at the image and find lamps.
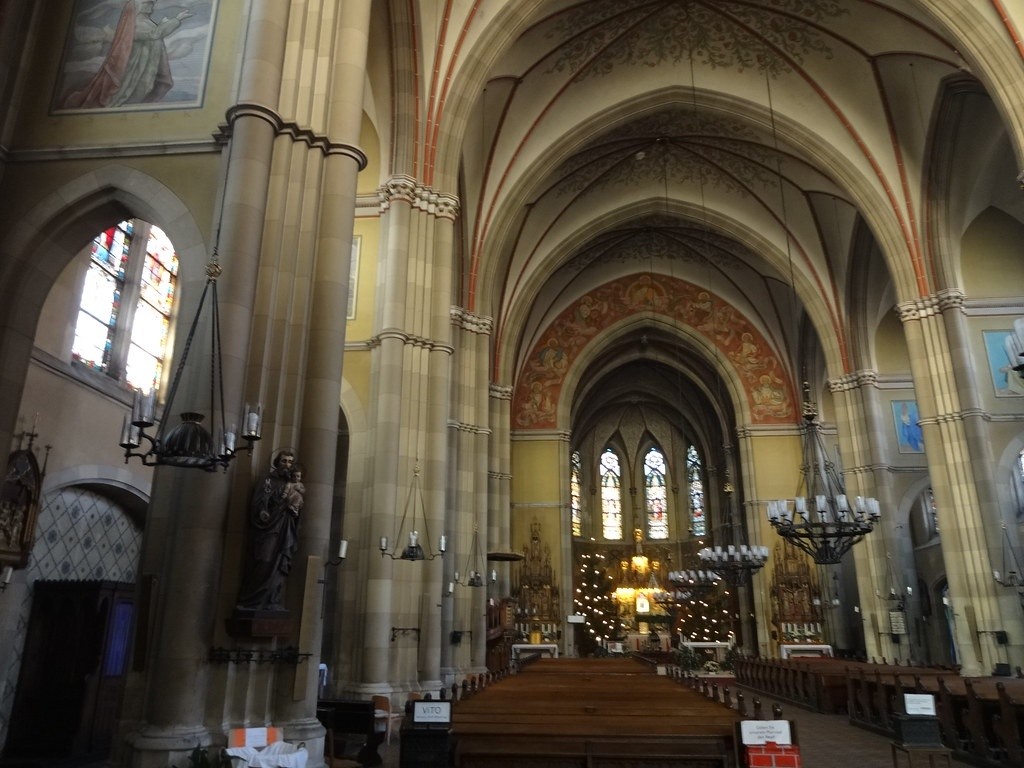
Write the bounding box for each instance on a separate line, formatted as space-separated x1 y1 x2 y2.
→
751 0 882 565
990 519 1024 587
686 8 769 587
812 564 841 608
651 138 722 616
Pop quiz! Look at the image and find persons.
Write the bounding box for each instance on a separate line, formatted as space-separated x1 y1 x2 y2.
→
280 468 305 518
236 449 305 615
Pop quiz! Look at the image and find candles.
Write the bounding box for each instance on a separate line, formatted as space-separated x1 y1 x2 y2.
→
448 582 454 593
876 586 912 597
247 412 259 435
513 584 558 635
410 533 417 545
943 596 947 605
454 567 497 583
339 537 349 557
855 606 859 612
440 536 445 550
120 387 156 446
381 537 387 550
489 597 494 605
221 431 236 456
781 621 822 642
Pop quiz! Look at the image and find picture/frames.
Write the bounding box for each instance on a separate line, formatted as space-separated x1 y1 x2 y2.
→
890 398 925 454
49 0 220 115
981 327 1024 398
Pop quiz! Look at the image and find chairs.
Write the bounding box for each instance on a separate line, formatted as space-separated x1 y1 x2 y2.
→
372 695 400 747
324 728 363 768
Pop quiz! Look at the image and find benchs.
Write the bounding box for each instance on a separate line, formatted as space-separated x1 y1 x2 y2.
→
400 653 801 768
731 651 1024 768
